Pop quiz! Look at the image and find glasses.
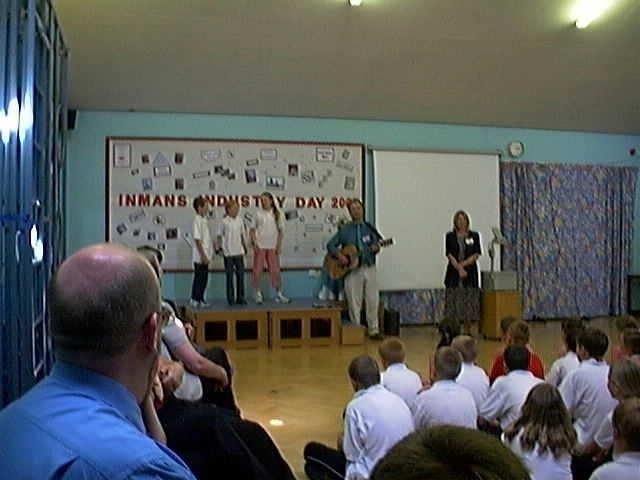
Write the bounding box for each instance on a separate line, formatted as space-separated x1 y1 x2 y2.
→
143 305 176 329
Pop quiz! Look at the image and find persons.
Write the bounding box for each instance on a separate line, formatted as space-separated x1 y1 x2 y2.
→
189 198 222 309
218 200 248 305
249 191 292 304
327 199 385 340
443 210 481 335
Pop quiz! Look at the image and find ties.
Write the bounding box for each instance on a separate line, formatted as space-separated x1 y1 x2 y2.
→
356 224 364 250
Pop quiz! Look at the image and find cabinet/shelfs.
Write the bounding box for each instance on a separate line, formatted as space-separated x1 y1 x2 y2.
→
190 301 269 351
270 306 346 348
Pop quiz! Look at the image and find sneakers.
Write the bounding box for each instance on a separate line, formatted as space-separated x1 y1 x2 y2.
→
189 292 291 304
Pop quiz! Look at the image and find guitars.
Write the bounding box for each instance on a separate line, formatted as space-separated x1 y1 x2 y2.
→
324 237 394 279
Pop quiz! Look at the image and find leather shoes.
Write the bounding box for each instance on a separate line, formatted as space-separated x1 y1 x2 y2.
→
369 332 384 340
